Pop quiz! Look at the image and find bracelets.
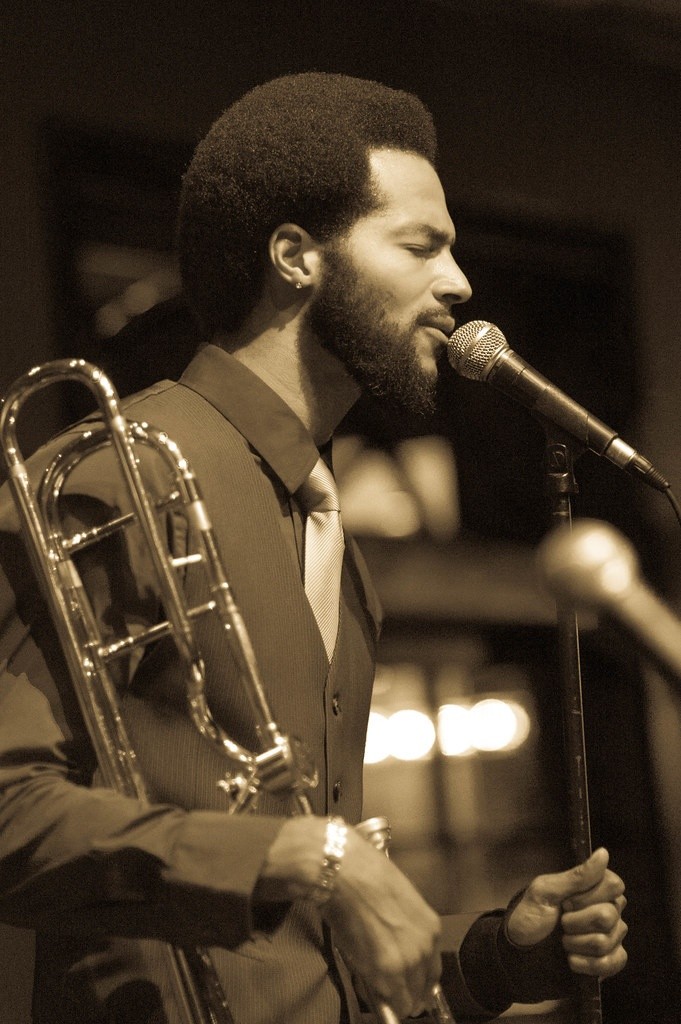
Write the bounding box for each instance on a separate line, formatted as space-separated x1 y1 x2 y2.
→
304 815 349 908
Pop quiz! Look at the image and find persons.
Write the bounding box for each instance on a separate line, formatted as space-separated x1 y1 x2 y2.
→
0 74 628 1024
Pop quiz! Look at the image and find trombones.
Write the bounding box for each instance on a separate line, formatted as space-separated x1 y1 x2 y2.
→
1 357 456 1024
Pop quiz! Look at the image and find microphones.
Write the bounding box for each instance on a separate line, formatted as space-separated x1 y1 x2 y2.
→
447 319 673 496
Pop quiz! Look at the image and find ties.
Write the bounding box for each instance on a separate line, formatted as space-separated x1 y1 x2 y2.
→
294 458 345 664
